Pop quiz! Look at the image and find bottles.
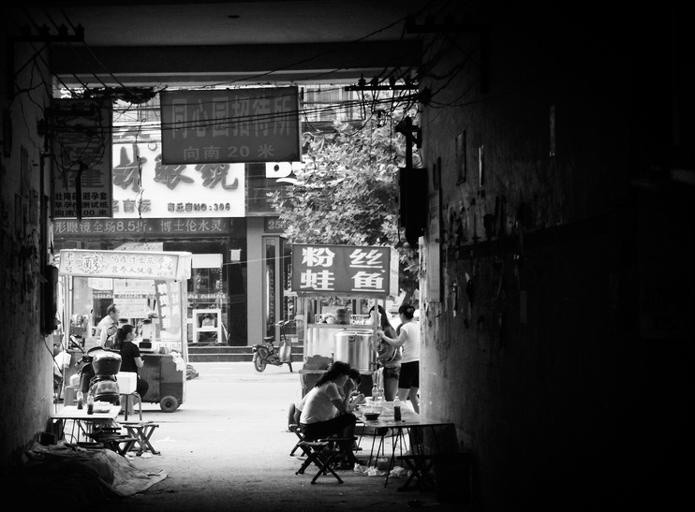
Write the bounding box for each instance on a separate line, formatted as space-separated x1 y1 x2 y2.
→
372 383 379 401
86 390 93 415
76 389 83 409
393 395 401 422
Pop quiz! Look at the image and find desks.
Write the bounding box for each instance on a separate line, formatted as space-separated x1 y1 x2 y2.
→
50 405 122 458
350 395 454 489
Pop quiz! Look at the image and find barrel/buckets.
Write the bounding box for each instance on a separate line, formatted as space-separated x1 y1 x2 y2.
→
333 329 374 379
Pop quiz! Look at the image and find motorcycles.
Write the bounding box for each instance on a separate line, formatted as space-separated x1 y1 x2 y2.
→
251 318 294 374
68 335 122 442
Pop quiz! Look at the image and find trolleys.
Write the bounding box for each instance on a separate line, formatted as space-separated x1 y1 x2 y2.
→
57 248 188 414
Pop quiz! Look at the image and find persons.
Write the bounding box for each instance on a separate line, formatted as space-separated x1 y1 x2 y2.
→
95 303 121 348
375 303 422 416
298 360 368 468
366 303 403 402
293 368 363 452
111 324 149 398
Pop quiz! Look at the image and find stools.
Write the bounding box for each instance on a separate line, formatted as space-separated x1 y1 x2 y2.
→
85 420 160 457
289 422 360 485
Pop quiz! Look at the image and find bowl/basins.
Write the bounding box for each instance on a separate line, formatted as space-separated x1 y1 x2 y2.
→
364 412 380 420
